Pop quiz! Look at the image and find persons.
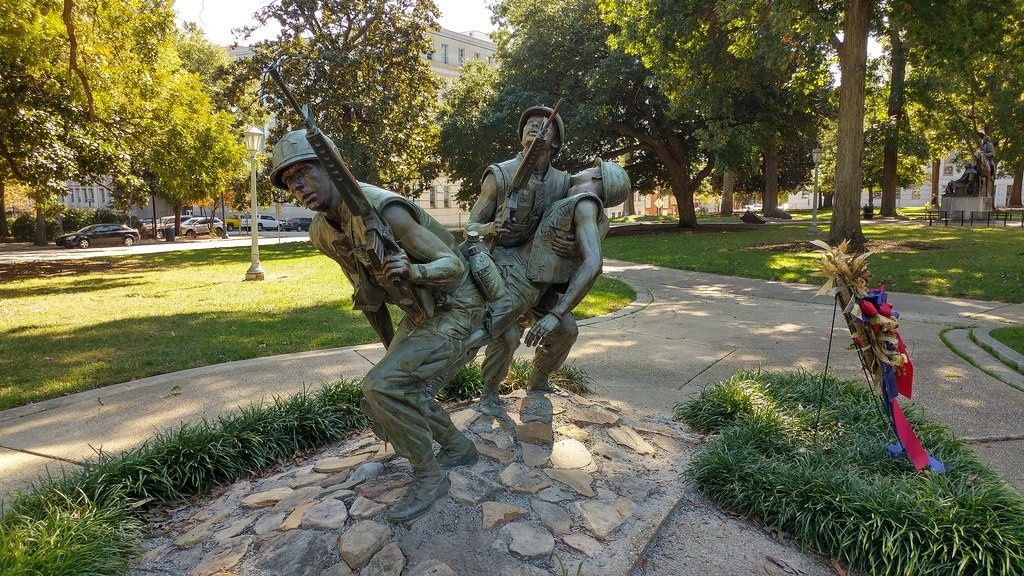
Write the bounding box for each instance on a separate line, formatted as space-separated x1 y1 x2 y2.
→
267 128 493 520
945 135 996 197
478 154 633 344
457 105 581 415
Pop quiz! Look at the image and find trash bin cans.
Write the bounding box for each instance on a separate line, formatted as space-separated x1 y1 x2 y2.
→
165 225 175 242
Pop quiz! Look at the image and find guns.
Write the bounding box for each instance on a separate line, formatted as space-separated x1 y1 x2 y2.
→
268 64 431 320
483 97 566 255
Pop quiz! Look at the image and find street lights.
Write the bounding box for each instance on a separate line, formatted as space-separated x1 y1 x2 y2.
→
243 125 266 273
812 146 822 232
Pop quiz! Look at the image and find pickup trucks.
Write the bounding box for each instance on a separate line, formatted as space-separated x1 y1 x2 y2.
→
225 213 244 232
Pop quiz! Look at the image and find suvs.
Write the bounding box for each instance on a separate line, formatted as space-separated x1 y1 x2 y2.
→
241 214 283 232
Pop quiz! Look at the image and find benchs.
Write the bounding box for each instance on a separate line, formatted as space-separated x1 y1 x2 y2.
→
860 212 880 219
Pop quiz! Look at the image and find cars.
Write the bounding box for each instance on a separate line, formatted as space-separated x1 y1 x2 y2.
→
283 218 312 232
56 223 139 248
140 215 223 238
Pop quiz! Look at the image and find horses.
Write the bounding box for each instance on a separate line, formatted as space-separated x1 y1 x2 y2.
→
974 148 997 199
